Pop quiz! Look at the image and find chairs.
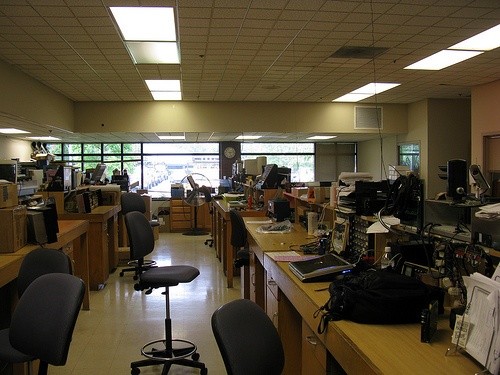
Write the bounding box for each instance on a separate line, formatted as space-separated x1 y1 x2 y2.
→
119 193 159 281
124 211 208 375
211 299 285 375
9 273 86 375
0 246 71 375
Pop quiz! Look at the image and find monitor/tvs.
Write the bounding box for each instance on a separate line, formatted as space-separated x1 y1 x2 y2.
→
446 160 469 202
91 164 106 182
47 166 71 192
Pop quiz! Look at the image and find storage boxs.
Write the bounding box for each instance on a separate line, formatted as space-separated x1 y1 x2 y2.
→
0 179 27 253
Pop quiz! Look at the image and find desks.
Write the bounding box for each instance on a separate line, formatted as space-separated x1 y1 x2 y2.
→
209 192 500 375
0 204 128 311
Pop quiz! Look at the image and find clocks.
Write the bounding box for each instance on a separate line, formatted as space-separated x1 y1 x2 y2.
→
223 147 236 159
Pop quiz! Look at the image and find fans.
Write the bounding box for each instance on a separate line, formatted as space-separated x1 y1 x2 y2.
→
179 173 212 235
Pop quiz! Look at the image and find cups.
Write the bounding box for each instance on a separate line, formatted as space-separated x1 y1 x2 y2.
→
308 212 318 235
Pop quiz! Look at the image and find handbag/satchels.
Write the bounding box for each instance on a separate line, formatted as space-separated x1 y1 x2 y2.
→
326 267 430 321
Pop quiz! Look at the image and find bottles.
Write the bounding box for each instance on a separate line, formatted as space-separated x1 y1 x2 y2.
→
330 182 338 207
381 247 394 268
248 195 252 208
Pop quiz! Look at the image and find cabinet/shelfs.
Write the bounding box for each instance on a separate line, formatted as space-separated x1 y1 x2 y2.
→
171 196 211 233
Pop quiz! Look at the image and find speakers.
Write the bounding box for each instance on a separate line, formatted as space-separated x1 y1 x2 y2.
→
0 159 17 184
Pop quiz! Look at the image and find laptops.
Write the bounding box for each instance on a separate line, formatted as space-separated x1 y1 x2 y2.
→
289 253 354 283
